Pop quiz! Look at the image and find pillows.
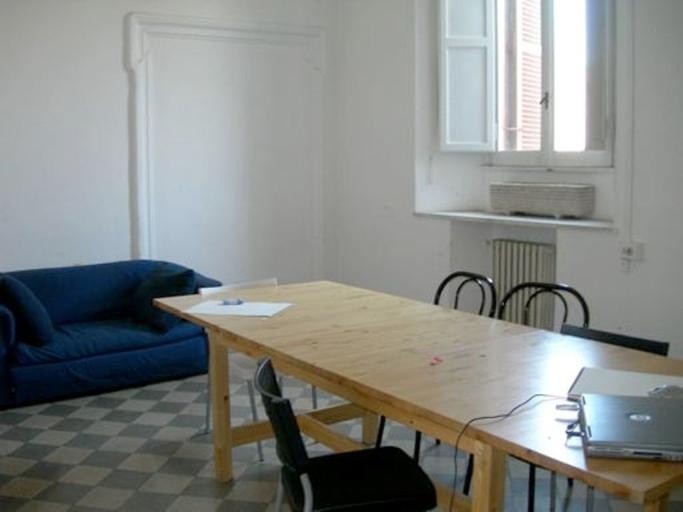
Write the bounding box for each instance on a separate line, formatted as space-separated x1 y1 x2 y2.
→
124 266 195 336
0 270 55 347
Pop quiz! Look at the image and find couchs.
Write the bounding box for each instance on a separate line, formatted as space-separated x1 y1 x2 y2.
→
1 258 222 410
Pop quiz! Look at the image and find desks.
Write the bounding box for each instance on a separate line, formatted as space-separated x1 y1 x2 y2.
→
151 280 682 511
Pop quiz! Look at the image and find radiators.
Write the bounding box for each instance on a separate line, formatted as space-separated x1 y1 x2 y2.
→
491 239 555 331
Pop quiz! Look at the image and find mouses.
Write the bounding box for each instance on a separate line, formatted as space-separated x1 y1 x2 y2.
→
647 384 683 398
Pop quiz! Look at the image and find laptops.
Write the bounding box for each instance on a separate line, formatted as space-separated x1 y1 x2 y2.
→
577 392 683 461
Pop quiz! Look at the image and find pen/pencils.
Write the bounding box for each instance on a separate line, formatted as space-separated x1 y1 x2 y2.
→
224 300 243 305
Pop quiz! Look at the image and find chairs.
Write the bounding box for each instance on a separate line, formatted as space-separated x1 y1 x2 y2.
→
377 270 499 467
462 280 587 510
255 356 437 512
